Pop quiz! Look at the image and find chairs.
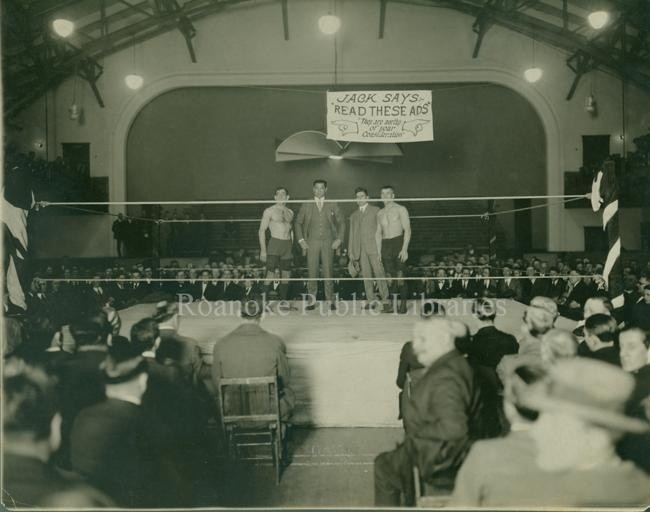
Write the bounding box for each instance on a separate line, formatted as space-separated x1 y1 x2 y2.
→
218 376 284 486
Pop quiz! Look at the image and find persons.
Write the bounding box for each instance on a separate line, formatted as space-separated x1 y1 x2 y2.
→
373 244 650 511
6 149 90 212
294 179 345 311
374 185 412 314
257 187 298 311
347 186 390 310
576 145 649 187
297 244 373 301
2 247 297 510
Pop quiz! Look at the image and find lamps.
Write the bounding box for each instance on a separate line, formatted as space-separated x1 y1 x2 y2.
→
125 33 142 91
524 30 542 87
318 0 342 35
588 2 609 30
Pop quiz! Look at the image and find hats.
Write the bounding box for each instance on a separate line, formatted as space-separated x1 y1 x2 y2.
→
100 343 148 384
526 356 649 436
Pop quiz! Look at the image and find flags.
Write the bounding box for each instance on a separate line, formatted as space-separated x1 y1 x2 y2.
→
326 88 434 143
